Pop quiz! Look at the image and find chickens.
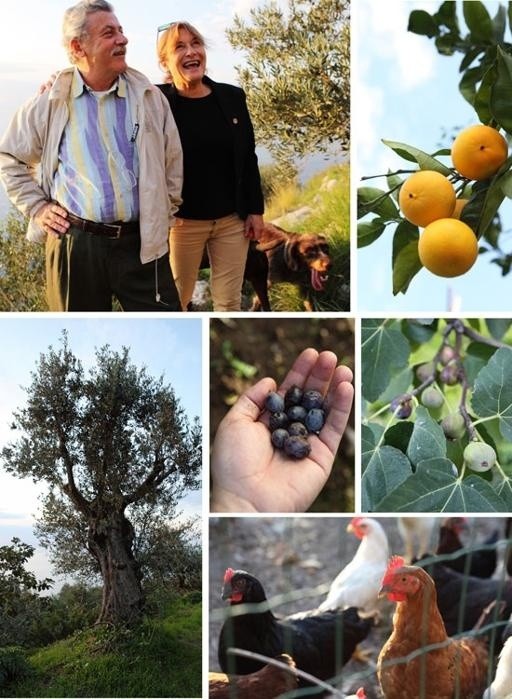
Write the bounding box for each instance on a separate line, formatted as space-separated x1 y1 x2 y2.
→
209 517 512 699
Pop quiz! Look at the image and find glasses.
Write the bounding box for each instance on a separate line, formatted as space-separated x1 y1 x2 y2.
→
157 22 176 42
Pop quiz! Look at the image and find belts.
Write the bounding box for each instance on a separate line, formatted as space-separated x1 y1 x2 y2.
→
66 213 140 240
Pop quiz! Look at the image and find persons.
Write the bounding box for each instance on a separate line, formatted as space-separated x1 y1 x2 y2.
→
0 0 197 312
207 347 354 512
141 19 267 312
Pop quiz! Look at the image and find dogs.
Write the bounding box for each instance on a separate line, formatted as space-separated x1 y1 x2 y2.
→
247 222 333 311
